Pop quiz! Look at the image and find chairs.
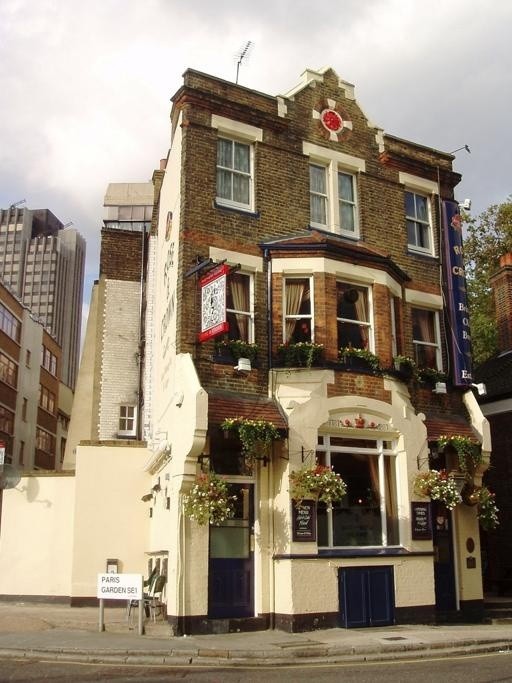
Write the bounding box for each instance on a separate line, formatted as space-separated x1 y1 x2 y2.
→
126 566 167 623
